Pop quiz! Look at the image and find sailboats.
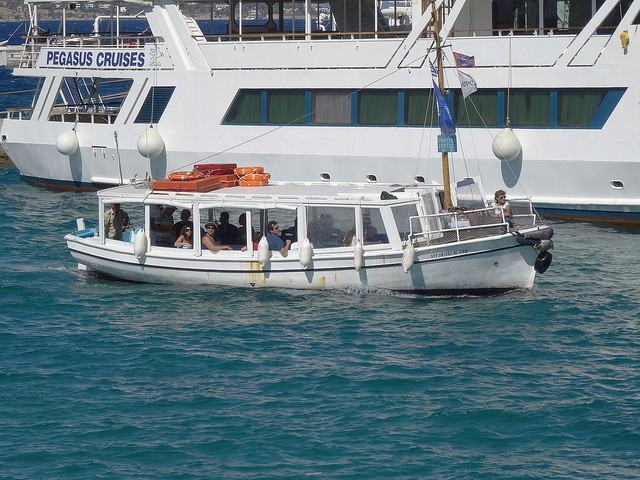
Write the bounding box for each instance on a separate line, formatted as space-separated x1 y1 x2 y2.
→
64 0 553 296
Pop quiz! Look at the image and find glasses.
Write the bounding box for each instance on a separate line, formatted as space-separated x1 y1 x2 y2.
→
500 196 506 200
186 229 192 232
207 226 215 230
273 226 279 230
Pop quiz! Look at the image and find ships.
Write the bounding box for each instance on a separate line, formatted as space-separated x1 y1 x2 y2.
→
0 0 640 226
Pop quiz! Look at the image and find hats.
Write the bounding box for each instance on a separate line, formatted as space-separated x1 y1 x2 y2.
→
205 222 219 231
363 213 373 223
219 212 229 220
165 206 177 210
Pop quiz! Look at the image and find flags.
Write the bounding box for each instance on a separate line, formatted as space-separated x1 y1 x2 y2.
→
453 52 475 68
433 80 456 140
458 70 478 98
429 57 438 79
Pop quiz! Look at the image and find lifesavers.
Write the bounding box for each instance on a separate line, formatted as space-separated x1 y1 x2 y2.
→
239 180 268 186
168 171 204 180
234 167 264 175
242 173 271 180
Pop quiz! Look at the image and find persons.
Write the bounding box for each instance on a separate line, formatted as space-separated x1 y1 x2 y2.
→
214 211 239 245
202 220 233 251
173 224 193 249
240 232 262 251
482 190 514 233
175 209 193 236
104 203 123 241
238 213 254 245
266 220 291 257
282 217 298 250
312 214 343 248
150 204 164 231
343 213 380 246
154 205 176 246
119 209 128 232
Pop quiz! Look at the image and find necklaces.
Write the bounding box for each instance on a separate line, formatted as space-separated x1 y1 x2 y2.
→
206 233 215 242
186 237 193 244
